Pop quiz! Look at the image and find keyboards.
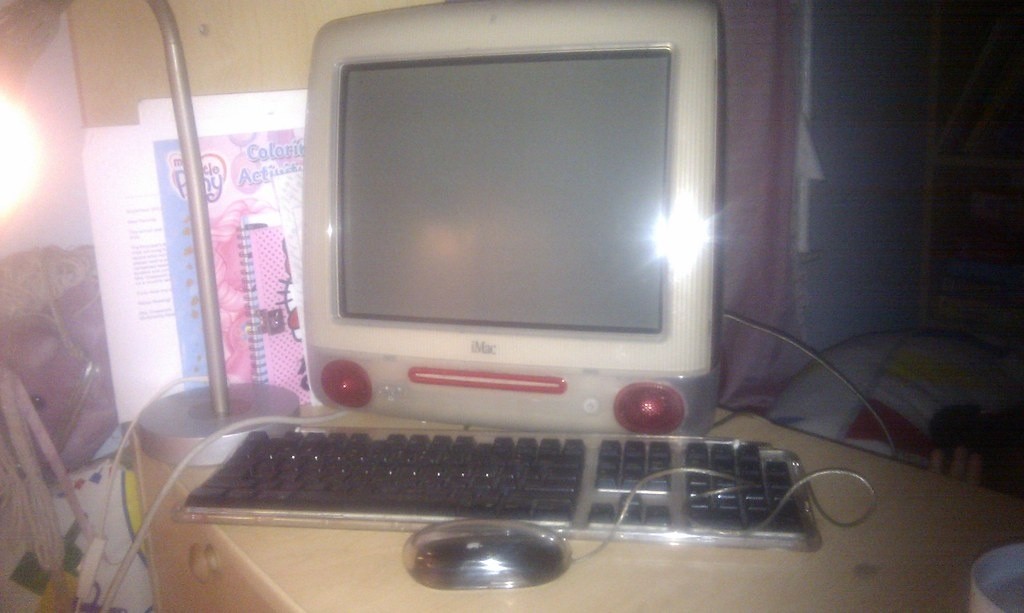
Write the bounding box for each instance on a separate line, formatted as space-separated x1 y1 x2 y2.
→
170 426 824 553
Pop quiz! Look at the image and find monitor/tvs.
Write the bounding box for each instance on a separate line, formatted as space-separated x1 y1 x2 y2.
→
304 0 725 433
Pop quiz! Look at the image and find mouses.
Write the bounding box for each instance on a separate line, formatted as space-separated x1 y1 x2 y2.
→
400 518 574 590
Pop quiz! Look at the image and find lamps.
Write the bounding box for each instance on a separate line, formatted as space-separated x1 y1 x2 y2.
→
0 1 300 468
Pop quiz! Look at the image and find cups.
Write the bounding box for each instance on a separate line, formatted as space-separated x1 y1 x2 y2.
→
965 546 1023 612
